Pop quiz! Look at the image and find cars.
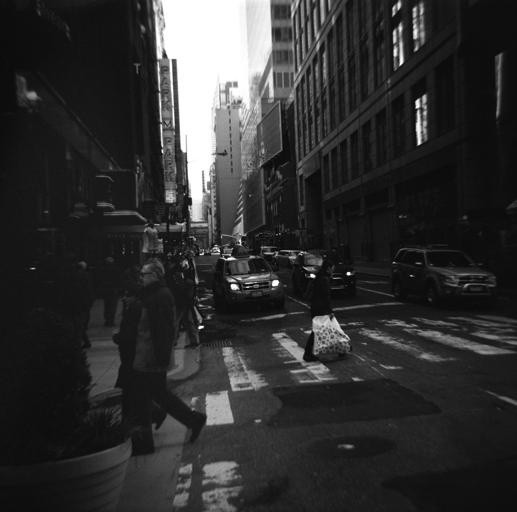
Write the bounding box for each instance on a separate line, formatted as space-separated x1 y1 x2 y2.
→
259 245 278 263
277 249 303 270
292 248 356 302
196 243 220 257
212 246 285 314
221 248 233 258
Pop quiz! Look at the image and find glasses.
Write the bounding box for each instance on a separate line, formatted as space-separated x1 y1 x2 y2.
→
141 271 152 277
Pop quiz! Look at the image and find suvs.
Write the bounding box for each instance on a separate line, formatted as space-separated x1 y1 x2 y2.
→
391 247 498 308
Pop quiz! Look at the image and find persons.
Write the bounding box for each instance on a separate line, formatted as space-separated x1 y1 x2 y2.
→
301 259 352 362
77 256 206 455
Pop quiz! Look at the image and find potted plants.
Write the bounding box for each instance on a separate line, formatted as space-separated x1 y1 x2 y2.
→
0 306 149 512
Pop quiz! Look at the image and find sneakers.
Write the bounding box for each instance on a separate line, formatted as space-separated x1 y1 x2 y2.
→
130 448 154 455
184 342 198 347
190 411 205 443
155 413 166 430
